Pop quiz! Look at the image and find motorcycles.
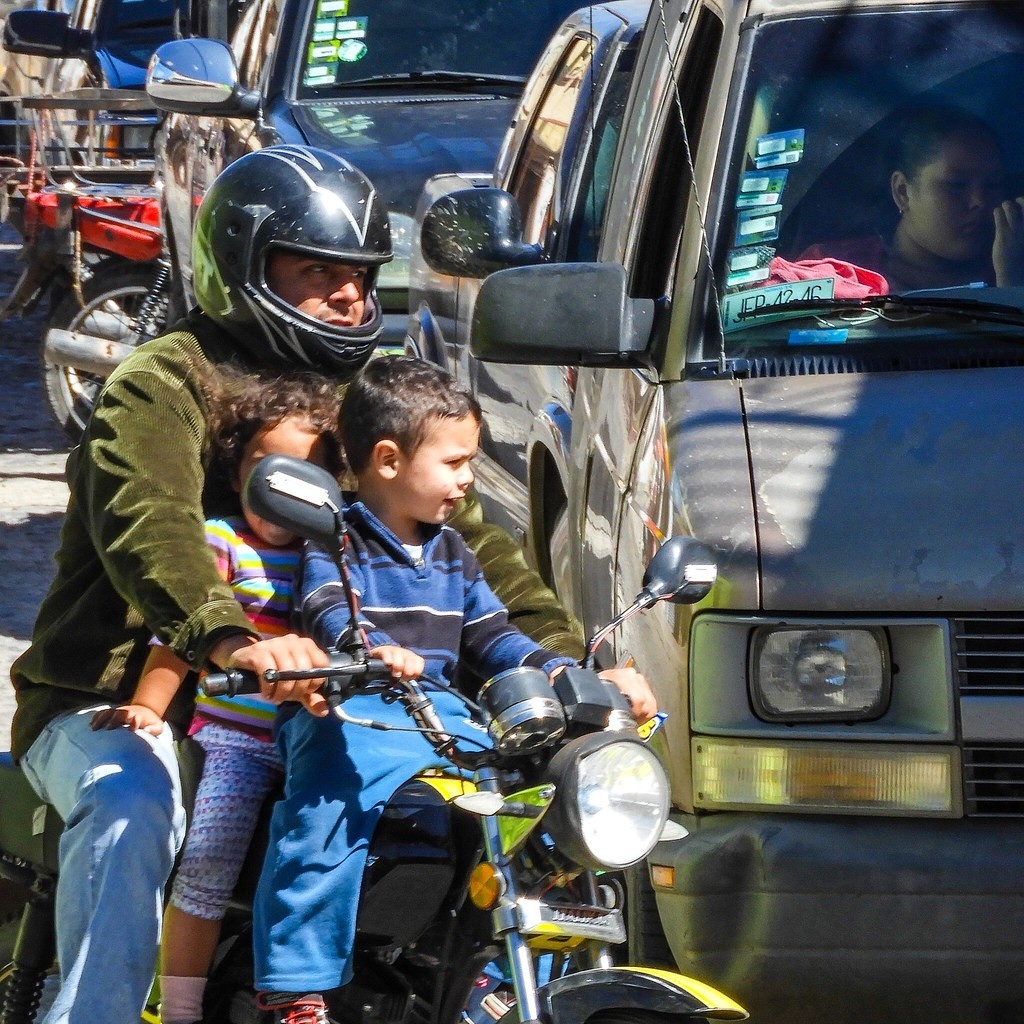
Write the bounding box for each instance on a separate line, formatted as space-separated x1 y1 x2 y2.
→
0 174 172 448
0 456 754 1024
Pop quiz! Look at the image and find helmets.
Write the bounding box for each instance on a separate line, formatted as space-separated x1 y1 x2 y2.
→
189 143 394 382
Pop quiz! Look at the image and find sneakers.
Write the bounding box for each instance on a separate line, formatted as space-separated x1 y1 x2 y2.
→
254 989 333 1024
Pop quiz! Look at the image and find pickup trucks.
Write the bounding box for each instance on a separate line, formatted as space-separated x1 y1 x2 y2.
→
403 0 656 652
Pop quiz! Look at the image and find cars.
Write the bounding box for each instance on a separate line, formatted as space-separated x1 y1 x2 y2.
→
2 0 253 164
147 0 616 363
470 0 1024 1024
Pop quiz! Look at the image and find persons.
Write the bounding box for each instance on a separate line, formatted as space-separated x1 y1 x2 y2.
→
795 111 1023 289
89 379 358 1024
249 355 660 1023
8 144 660 1024
